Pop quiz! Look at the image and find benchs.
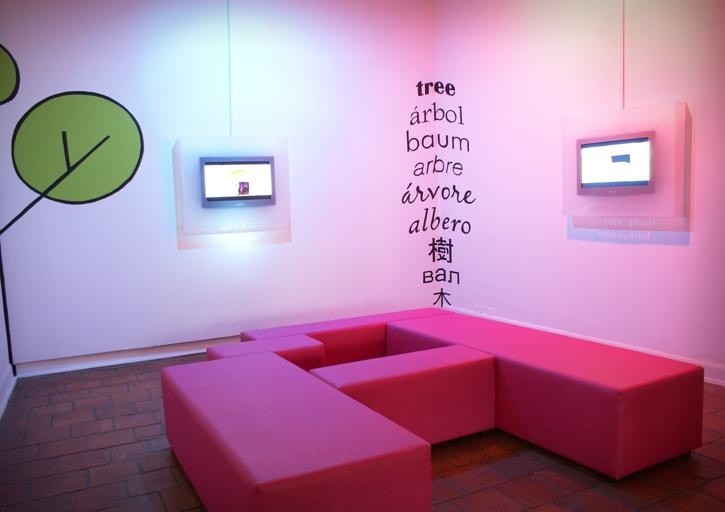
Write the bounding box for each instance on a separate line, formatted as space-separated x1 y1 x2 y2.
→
308 344 496 445
237 306 459 368
385 313 706 480
203 334 326 371
157 352 433 511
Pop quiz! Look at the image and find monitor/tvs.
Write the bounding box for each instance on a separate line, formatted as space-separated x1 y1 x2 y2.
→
200 156 276 208
576 130 656 197
